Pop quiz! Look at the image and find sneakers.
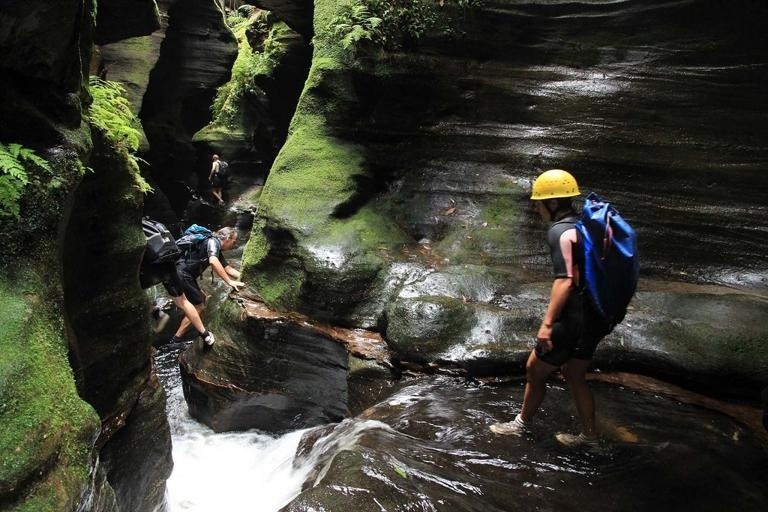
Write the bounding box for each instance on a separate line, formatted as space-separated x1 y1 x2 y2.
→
219 200 226 206
555 431 603 454
489 420 532 438
205 330 215 345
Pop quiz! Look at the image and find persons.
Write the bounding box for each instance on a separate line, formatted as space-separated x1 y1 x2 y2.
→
489 168 609 449
169 223 246 349
208 154 225 206
140 219 216 346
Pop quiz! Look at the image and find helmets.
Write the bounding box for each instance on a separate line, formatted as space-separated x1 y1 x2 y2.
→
530 169 580 201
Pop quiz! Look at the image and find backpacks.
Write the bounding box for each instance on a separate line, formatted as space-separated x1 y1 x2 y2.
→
176 223 211 253
215 160 229 177
142 217 180 275
577 194 640 320
